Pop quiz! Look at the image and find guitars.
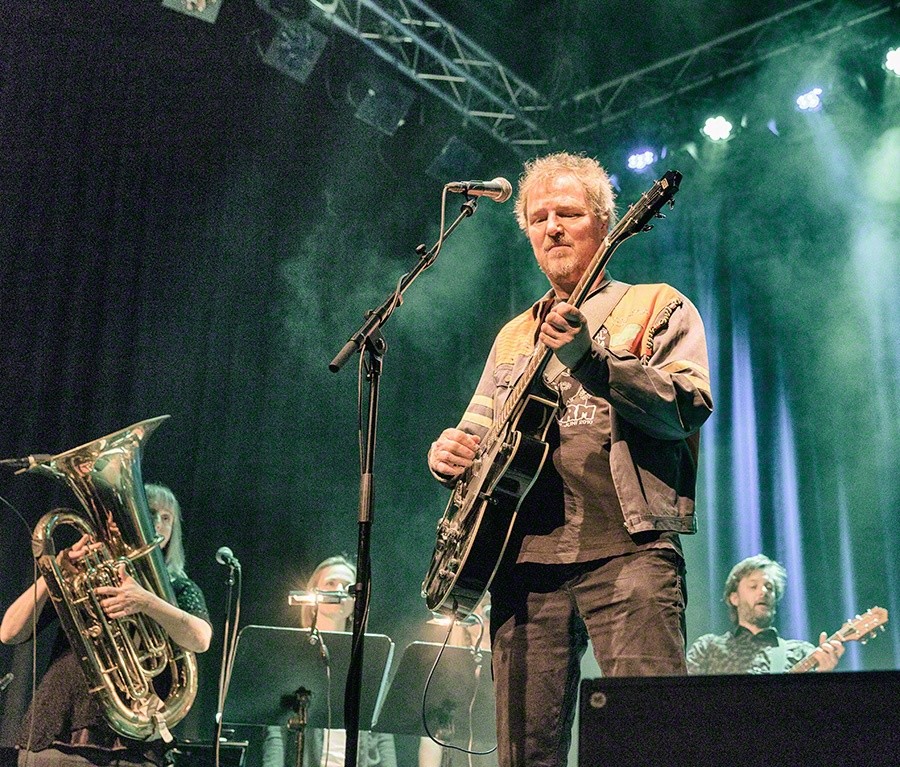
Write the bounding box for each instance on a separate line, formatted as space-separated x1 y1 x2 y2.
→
784 607 891 673
419 169 685 621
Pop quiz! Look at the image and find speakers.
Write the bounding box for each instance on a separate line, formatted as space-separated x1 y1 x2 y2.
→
577 668 900 767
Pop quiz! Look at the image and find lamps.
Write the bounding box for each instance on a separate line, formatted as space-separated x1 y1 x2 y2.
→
426 130 483 190
348 68 414 136
161 0 223 25
251 7 329 83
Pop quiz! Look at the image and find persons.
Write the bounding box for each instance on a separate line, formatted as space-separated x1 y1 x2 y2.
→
416 588 500 767
0 483 214 767
427 153 715 767
685 556 846 673
263 557 401 767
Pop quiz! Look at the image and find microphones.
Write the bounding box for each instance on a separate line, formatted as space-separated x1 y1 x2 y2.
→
7 453 52 464
444 176 513 203
215 546 240 568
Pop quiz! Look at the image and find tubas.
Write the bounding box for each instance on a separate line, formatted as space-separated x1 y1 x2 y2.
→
13 414 197 740
